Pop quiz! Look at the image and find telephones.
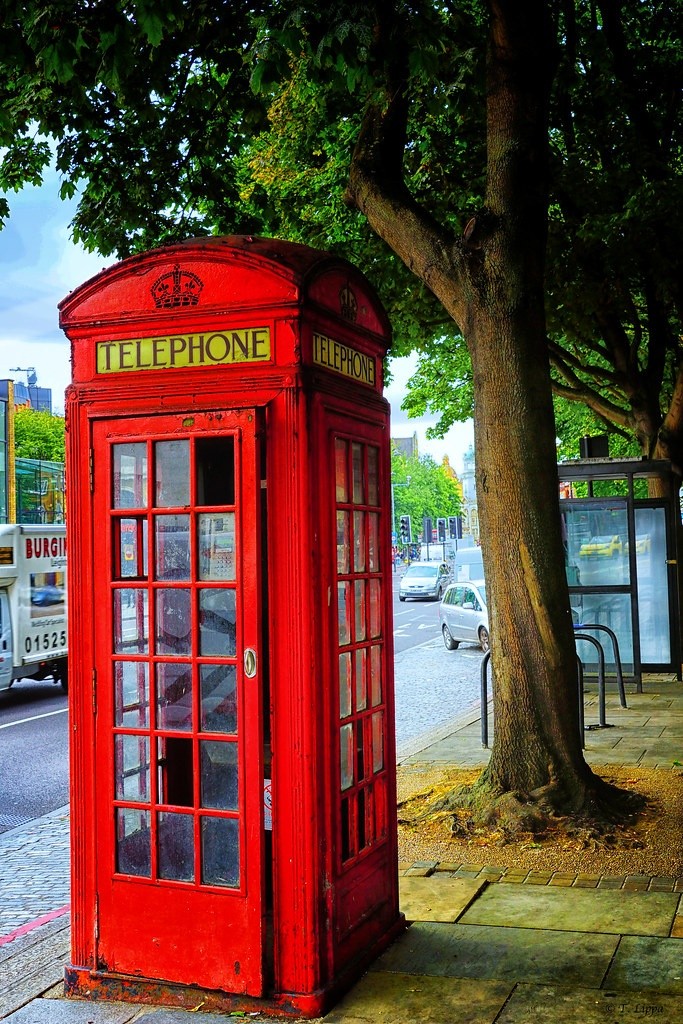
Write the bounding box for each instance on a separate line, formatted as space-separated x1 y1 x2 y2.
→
197 512 236 581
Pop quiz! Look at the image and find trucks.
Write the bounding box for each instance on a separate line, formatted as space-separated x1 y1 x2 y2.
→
0 524 68 690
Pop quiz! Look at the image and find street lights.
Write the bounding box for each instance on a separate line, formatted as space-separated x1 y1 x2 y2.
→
391 470 412 572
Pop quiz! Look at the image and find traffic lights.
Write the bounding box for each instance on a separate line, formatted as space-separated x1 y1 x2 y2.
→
400 515 412 545
437 518 446 542
449 517 462 539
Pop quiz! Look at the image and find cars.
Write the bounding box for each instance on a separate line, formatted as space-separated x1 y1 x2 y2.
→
399 561 451 601
624 534 651 557
439 580 489 653
579 535 623 561
